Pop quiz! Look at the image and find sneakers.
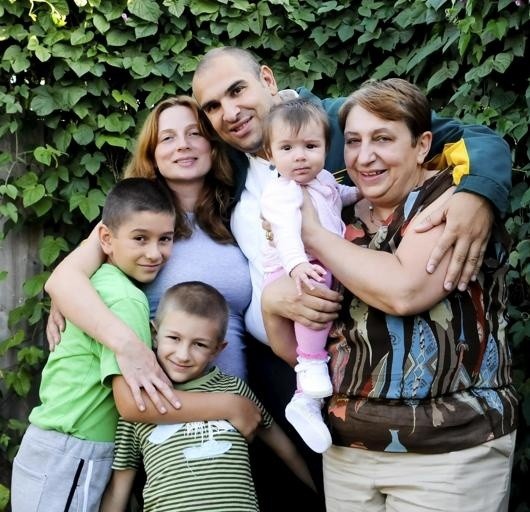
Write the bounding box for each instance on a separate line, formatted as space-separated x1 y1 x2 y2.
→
285 394 332 454
294 363 333 399
